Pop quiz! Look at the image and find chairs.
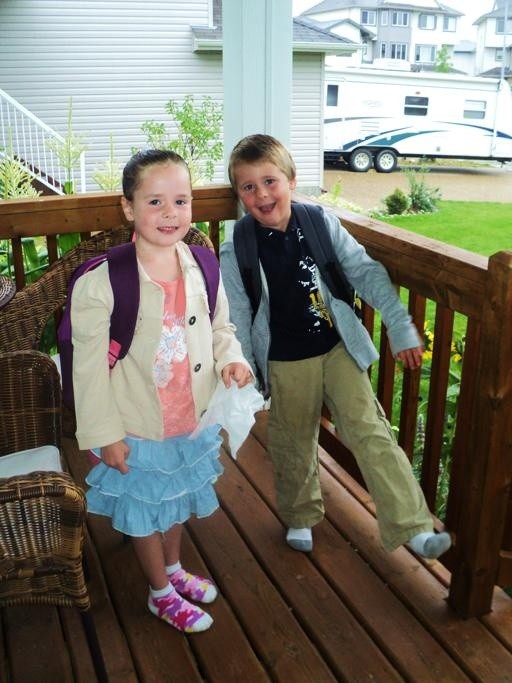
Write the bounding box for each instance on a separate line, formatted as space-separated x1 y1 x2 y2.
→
1 217 220 355
0 348 93 612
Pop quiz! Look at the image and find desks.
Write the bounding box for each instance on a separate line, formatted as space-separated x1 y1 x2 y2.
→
1 274 17 309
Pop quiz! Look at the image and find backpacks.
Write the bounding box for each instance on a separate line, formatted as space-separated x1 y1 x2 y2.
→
53 240 222 439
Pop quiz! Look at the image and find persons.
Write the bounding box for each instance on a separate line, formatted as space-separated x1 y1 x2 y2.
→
216 131 454 560
66 146 258 637
